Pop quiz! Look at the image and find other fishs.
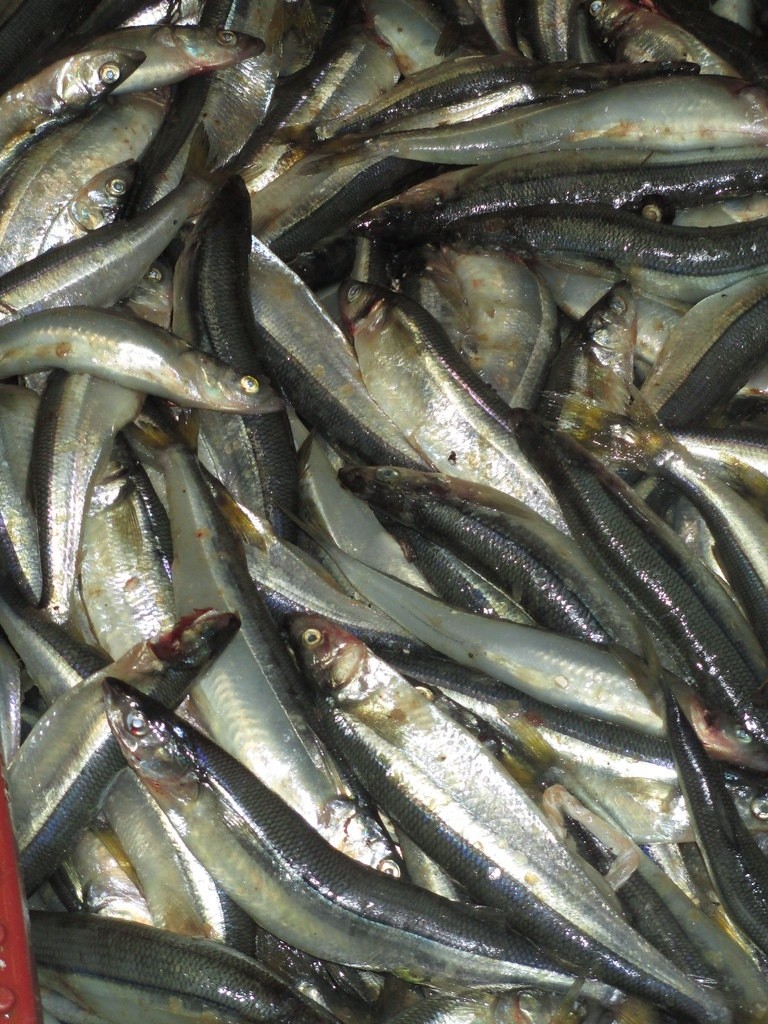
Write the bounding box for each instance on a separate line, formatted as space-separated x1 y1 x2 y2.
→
0 1 768 1024
